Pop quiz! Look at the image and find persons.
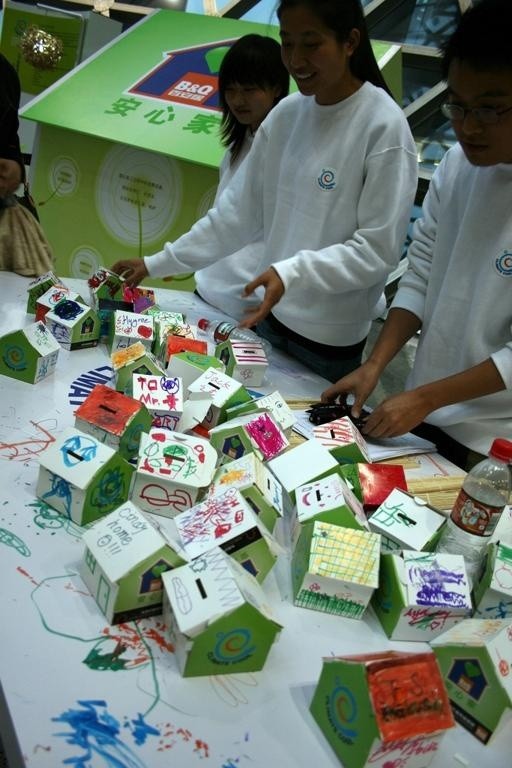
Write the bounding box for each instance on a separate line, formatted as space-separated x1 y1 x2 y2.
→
0 52 26 216
321 0 512 478
191 34 291 331
110 0 419 385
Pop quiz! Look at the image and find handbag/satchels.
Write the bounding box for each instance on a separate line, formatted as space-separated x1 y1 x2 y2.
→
4 153 39 224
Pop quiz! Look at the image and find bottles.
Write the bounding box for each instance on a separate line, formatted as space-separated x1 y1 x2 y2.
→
433 436 512 594
197 317 273 360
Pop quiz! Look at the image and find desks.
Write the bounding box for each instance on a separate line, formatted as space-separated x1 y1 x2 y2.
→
0 273 512 768
